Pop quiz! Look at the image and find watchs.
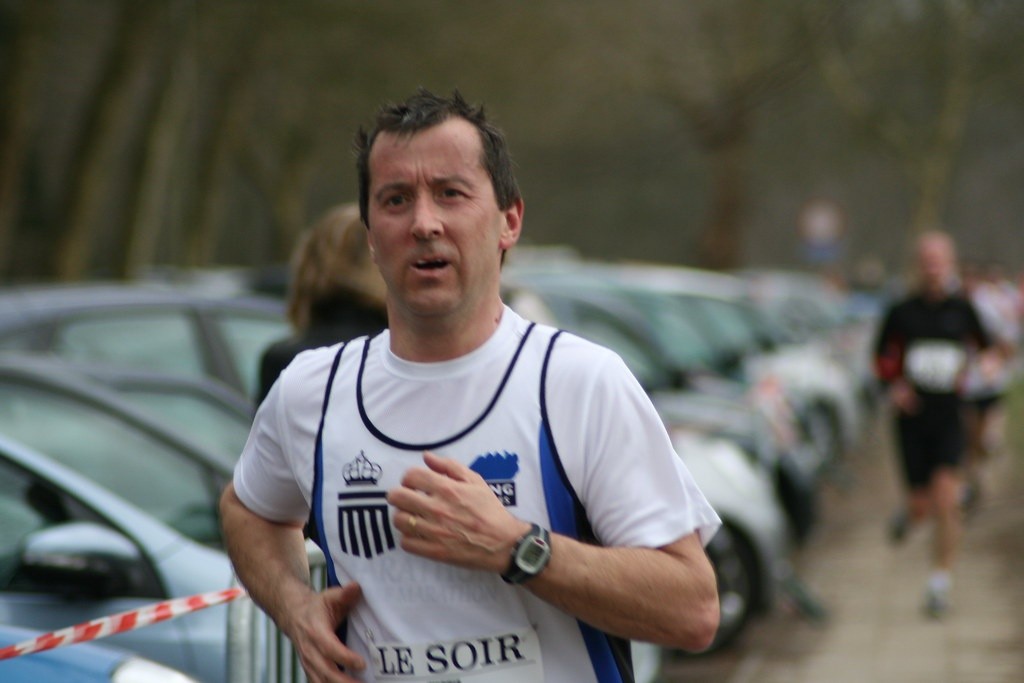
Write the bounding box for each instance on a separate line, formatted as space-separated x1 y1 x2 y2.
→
499 524 553 585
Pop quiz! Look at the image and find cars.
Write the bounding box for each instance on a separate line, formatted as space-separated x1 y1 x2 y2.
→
1 250 892 682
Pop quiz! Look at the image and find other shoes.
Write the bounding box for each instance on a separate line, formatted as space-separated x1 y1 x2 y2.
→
921 582 945 619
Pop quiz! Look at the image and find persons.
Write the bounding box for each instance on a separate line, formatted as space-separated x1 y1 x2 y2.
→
873 230 1024 616
257 203 388 404
219 83 720 683
